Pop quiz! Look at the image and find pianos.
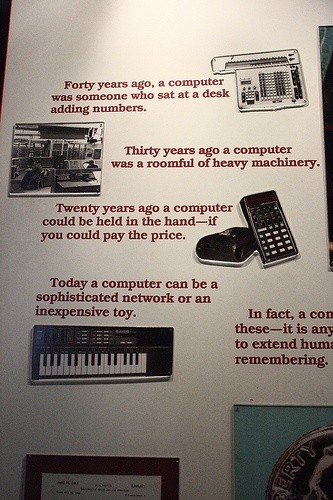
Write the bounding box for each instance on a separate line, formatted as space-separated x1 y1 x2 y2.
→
27 324 175 385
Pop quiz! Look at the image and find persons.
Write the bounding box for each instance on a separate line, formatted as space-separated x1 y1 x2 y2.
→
86 161 99 169
22 161 42 192
